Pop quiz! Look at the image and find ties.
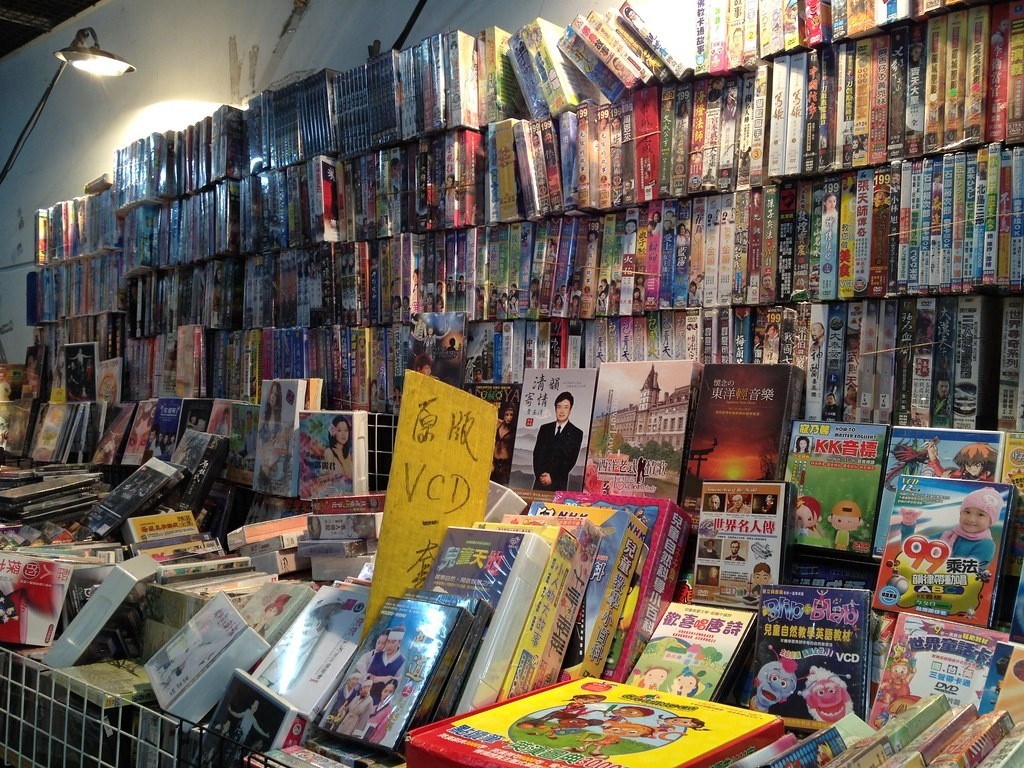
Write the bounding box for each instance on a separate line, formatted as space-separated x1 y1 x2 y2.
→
556 426 561 435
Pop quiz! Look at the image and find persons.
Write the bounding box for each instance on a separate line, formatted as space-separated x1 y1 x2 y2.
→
2 2 1022 745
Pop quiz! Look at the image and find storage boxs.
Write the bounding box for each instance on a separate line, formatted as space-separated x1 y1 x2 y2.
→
42 359 1024 768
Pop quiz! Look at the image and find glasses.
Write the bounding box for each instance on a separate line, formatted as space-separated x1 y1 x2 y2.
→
732 546 739 548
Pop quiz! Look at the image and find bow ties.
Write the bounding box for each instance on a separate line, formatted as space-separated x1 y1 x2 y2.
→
731 556 737 558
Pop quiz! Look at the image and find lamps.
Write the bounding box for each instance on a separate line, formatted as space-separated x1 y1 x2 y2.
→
0 26 137 186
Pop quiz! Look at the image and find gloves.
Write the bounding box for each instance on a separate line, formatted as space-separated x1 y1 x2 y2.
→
901 507 922 525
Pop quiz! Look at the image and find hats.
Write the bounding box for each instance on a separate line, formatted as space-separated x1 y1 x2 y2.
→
704 539 714 546
961 487 1004 525
